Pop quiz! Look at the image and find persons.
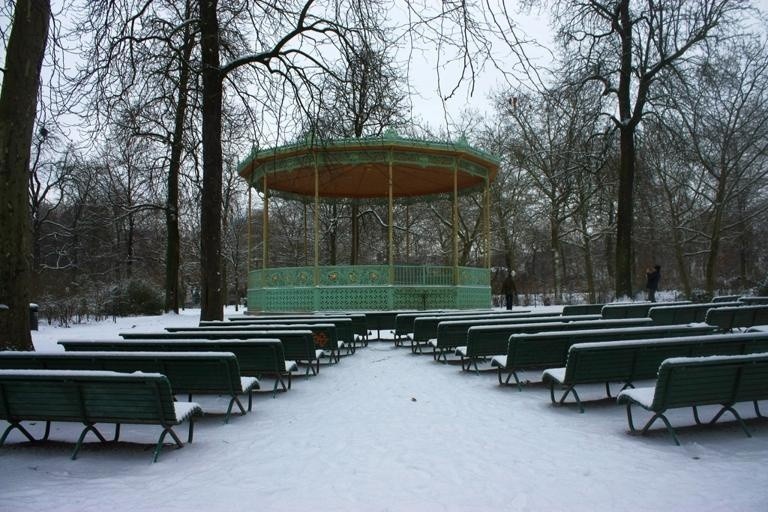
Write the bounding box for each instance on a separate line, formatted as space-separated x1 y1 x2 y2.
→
646 266 661 303
501 274 517 310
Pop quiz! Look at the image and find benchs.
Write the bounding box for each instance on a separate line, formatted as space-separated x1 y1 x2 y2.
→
540 332 767 412
391 295 767 392
0 311 377 398
1 370 203 463
616 352 767 448
0 350 260 422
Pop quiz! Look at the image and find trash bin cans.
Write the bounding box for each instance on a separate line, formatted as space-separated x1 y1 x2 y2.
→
29 303 39 332
